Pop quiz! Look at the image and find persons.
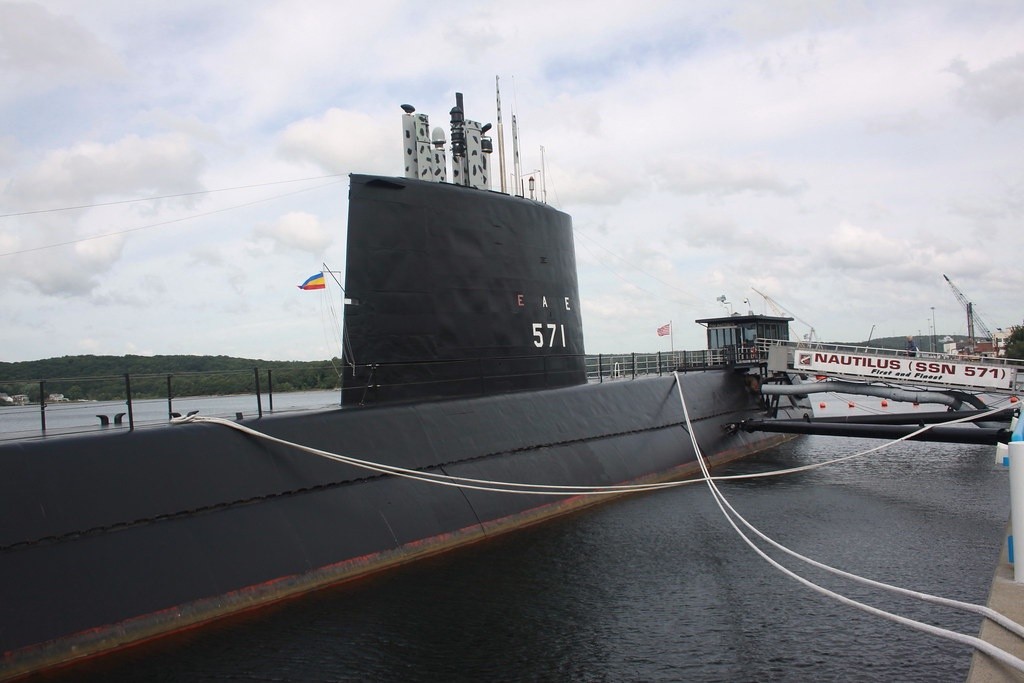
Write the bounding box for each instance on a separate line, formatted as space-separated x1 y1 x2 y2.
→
907 336 917 357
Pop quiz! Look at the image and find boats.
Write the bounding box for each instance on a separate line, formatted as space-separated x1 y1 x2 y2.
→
0 75 814 683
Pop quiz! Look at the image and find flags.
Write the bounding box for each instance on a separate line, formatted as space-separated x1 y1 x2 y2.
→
657 324 670 336
298 272 325 290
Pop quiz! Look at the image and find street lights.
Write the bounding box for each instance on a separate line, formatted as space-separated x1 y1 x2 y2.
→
917 306 939 353
